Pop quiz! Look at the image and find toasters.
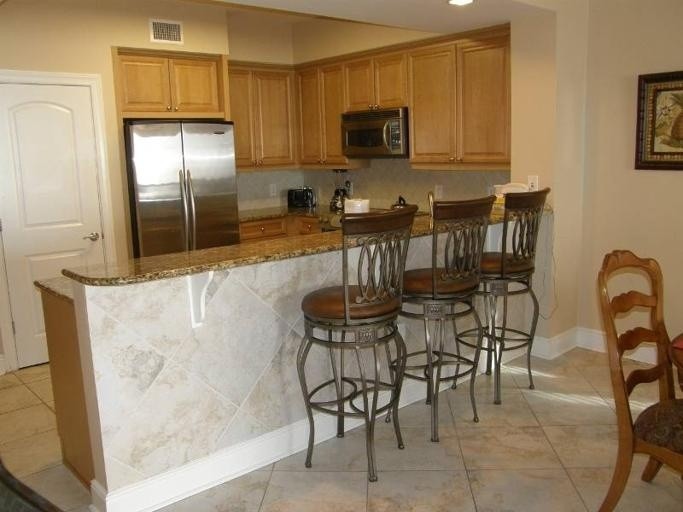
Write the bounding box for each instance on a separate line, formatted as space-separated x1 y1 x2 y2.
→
287 186 316 207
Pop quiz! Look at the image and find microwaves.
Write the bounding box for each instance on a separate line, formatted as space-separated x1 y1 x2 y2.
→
339 106 410 160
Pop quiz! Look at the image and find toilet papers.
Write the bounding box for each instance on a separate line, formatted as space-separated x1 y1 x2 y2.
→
490 181 534 219
343 198 370 219
434 184 442 200
526 173 539 194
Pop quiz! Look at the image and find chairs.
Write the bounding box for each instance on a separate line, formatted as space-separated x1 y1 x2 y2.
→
448 186 551 404
295 204 418 482
596 247 683 511
385 193 496 442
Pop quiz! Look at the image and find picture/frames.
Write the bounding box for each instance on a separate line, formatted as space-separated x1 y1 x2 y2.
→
634 70 683 171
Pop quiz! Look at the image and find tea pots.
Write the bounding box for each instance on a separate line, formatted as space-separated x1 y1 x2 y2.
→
390 195 409 210
328 188 349 212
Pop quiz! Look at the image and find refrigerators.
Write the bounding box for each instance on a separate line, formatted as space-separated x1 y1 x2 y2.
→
122 114 243 259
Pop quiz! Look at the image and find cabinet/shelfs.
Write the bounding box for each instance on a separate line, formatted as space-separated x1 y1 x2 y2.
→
112 46 226 119
291 56 370 171
228 63 295 173
284 215 323 238
239 216 285 245
408 24 510 172
345 48 409 112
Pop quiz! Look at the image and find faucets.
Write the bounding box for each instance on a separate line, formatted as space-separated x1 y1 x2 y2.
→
303 186 316 207
